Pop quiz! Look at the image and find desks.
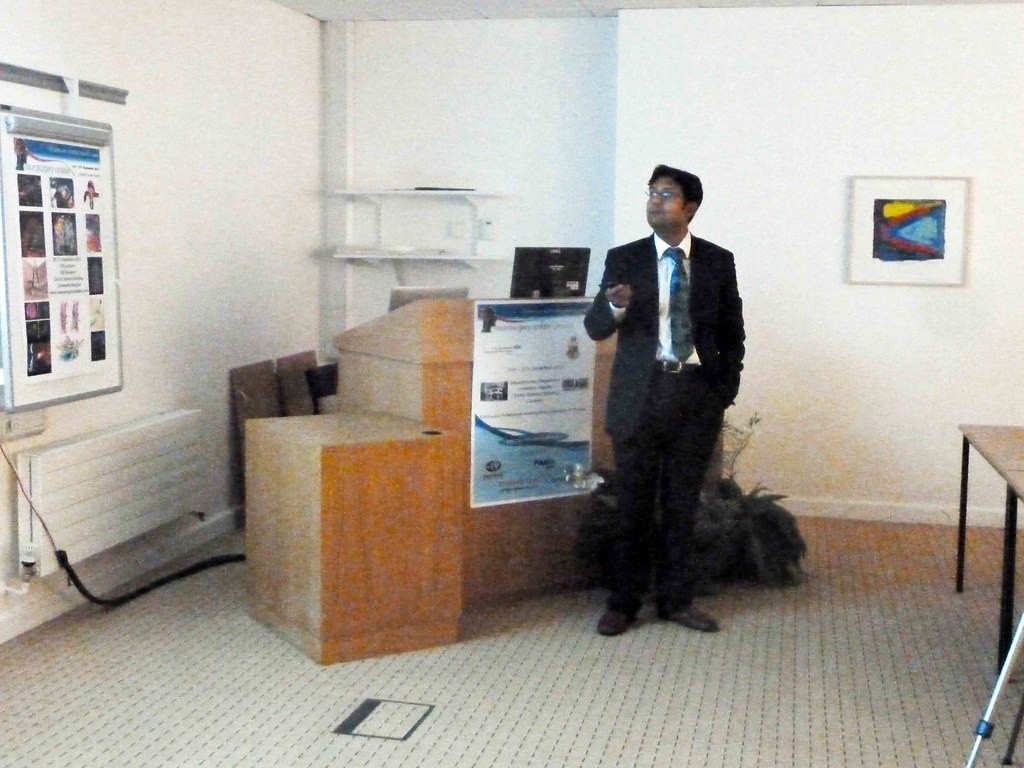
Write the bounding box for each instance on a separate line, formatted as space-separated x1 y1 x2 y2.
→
954 423 1024 676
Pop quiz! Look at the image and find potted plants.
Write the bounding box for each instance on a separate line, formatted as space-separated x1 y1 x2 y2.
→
577 414 807 600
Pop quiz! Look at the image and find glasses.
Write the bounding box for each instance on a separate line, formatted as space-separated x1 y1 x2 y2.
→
646 190 685 200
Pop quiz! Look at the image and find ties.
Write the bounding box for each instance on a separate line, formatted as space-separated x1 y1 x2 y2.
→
664 247 696 363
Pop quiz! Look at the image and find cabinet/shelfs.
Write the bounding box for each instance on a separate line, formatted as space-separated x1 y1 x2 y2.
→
334 187 505 269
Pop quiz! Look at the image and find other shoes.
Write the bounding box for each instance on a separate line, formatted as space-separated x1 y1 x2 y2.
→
655 600 718 631
597 606 633 635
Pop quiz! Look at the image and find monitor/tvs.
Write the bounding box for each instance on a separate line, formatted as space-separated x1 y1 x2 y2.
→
511 246 591 300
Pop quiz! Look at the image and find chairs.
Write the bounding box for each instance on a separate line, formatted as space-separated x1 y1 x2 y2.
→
228 348 340 461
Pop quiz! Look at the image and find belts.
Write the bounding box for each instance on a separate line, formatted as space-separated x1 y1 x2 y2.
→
653 360 704 376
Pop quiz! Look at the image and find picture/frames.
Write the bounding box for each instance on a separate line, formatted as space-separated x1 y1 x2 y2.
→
842 176 973 287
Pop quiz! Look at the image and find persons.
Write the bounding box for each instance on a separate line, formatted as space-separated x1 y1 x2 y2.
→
583 166 747 635
